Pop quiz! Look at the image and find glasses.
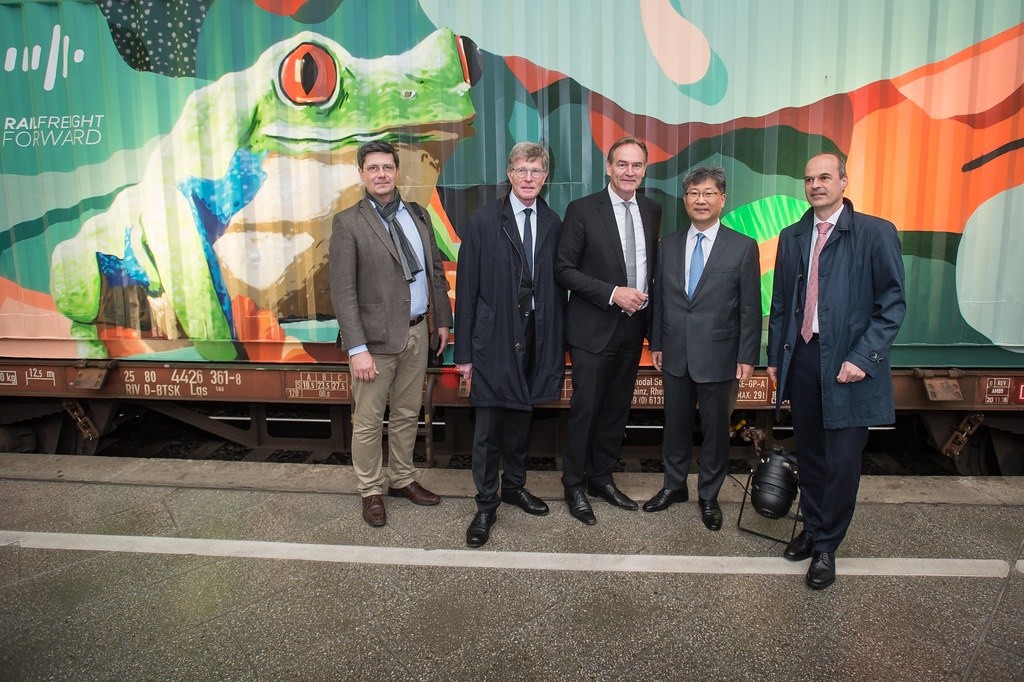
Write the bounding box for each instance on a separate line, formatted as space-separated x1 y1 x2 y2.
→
362 165 396 172
511 168 545 179
686 192 724 198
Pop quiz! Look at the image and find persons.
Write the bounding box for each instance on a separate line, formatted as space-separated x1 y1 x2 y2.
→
766 152 906 589
453 141 570 550
553 135 665 526
641 168 762 530
332 139 453 527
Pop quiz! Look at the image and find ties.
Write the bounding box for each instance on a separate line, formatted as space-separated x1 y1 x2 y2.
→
801 222 833 344
522 208 533 280
622 202 636 317
688 233 706 300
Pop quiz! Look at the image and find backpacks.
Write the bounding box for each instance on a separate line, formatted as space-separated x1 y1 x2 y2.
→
428 337 444 367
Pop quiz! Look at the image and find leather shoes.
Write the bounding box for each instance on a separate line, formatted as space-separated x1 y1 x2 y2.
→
466 509 497 546
587 482 638 510
361 494 386 525
501 487 550 516
783 529 815 561
388 481 440 506
698 495 723 532
643 486 689 512
564 487 596 524
806 550 837 590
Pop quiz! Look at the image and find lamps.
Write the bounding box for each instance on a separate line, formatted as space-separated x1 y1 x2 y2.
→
737 445 803 546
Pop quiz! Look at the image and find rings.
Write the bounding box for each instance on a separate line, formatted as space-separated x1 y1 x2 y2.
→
851 379 856 383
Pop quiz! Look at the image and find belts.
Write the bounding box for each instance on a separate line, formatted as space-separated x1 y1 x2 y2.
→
409 312 427 327
